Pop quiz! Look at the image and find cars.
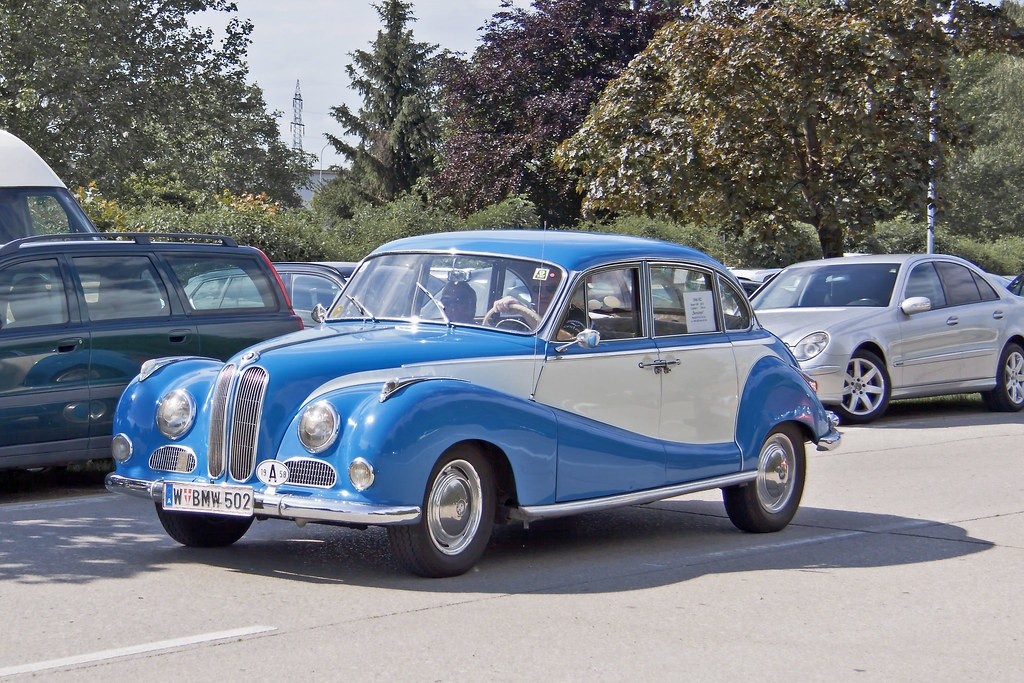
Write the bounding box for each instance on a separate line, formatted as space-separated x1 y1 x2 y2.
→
314 264 674 319
693 252 1024 426
104 227 843 581
177 262 349 329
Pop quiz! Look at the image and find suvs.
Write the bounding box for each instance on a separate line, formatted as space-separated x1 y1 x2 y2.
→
0 230 304 472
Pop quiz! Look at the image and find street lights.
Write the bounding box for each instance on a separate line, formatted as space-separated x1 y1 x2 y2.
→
318 135 343 192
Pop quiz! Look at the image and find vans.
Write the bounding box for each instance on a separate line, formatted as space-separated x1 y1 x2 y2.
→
0 127 165 325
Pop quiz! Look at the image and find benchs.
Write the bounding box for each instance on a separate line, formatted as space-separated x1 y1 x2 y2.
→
96 280 161 317
589 311 740 337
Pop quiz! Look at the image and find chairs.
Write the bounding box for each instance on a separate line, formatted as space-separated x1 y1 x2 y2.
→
7 277 52 326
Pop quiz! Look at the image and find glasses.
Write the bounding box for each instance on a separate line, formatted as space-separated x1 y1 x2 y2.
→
534 284 558 293
441 297 468 305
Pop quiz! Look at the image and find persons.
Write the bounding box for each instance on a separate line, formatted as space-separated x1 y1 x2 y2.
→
441 281 476 322
491 273 603 341
